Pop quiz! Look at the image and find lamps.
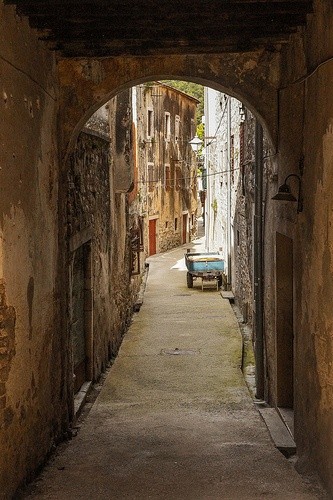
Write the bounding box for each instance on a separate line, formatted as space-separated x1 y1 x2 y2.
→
270 174 304 215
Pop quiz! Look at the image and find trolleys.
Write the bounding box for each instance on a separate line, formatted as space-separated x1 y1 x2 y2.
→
185 247 225 293
